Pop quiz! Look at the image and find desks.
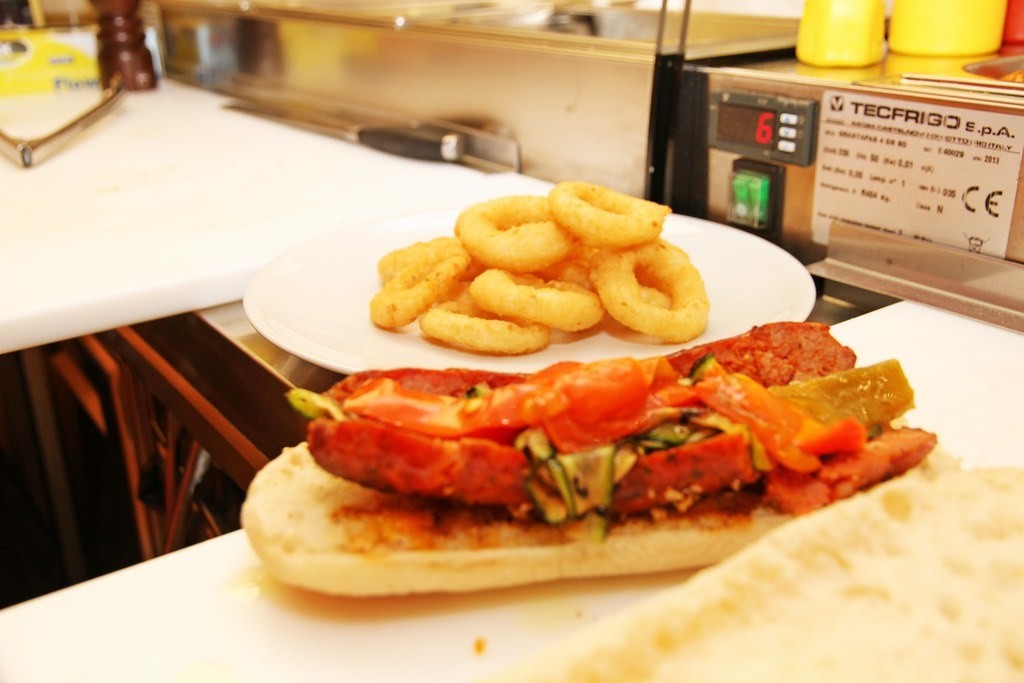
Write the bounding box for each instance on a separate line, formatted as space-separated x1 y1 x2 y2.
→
0 297 1024 683
0 62 483 358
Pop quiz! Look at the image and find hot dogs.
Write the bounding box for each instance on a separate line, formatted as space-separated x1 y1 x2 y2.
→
237 319 959 596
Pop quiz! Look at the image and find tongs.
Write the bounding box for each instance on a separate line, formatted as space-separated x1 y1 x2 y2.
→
1 84 124 165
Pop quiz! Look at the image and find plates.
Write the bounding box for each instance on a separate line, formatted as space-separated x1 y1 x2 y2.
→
242 199 817 378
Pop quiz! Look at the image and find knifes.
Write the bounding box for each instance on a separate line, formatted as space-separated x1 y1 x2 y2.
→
225 106 464 162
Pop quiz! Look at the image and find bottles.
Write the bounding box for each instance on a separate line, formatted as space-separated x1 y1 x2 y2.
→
88 0 155 92
